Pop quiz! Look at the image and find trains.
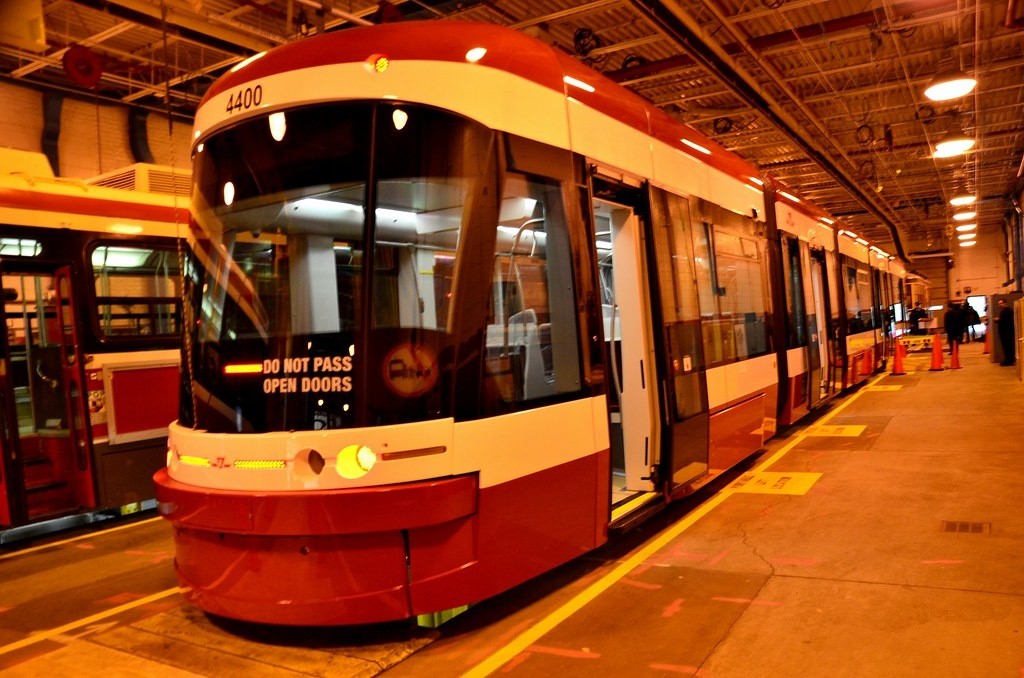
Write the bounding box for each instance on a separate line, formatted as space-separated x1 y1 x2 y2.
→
0 147 198 528
144 19 931 628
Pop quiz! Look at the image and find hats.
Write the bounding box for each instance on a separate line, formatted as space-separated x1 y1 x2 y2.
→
915 301 922 306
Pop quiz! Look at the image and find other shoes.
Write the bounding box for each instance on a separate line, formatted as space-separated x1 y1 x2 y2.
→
999 361 1013 366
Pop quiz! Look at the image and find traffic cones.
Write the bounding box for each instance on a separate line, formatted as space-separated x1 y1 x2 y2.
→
889 337 911 375
981 327 992 353
926 332 946 371
945 341 964 370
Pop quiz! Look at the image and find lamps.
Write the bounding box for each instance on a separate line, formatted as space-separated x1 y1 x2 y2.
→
950 183 978 221
932 109 976 157
923 40 978 100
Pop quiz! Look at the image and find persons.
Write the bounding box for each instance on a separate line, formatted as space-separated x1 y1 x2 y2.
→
994 299 1016 366
944 302 963 354
955 302 980 342
909 301 928 336
850 311 864 333
